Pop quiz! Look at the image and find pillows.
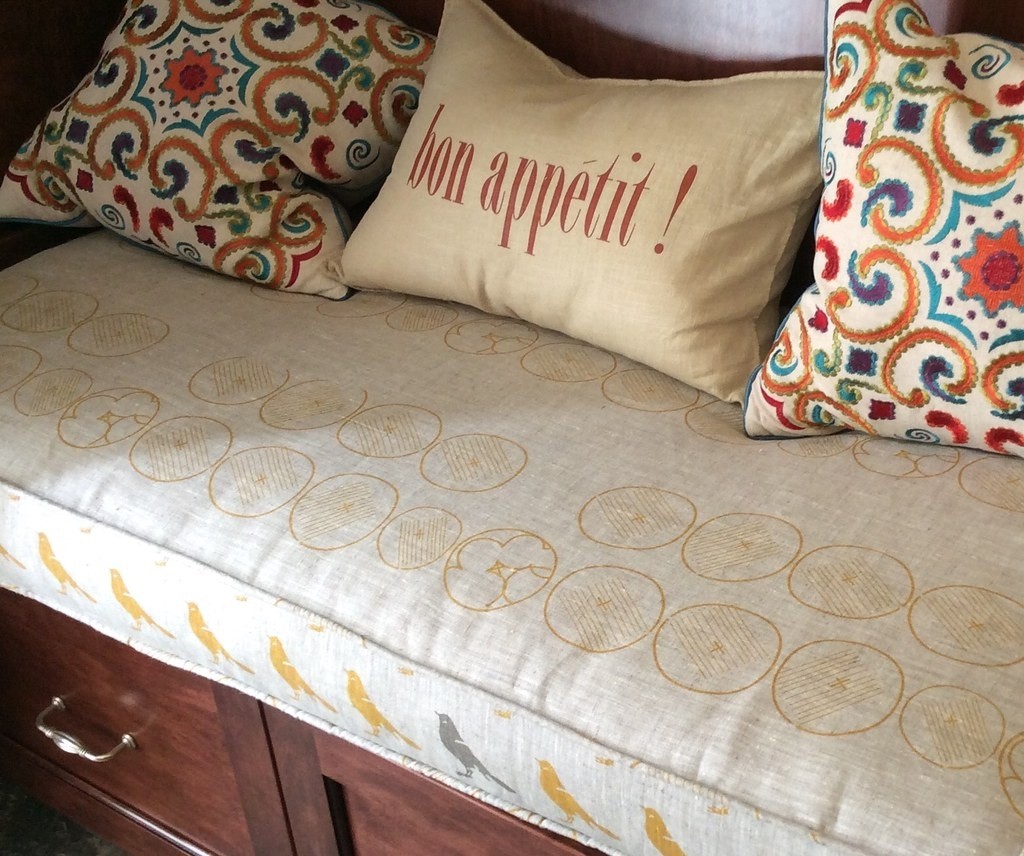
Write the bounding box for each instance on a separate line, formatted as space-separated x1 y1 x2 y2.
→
0 0 439 294
320 0 836 405
742 3 1023 458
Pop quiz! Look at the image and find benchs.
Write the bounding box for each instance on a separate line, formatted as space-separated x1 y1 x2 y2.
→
1 220 1021 856
